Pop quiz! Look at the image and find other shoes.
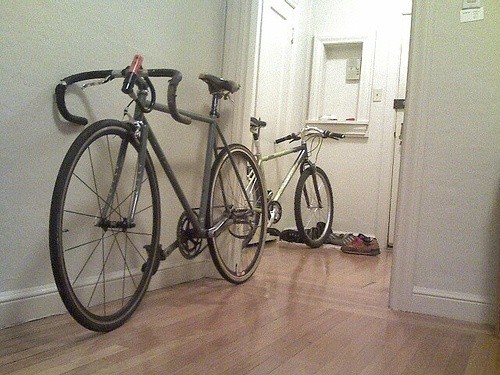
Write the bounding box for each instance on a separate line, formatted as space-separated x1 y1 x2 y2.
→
318 221 342 244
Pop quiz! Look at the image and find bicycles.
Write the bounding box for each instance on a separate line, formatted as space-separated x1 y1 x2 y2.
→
241 116 346 250
46 55 269 332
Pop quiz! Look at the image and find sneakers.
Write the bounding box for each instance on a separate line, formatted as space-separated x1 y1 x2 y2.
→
341 234 381 257
279 229 311 244
334 233 357 246
